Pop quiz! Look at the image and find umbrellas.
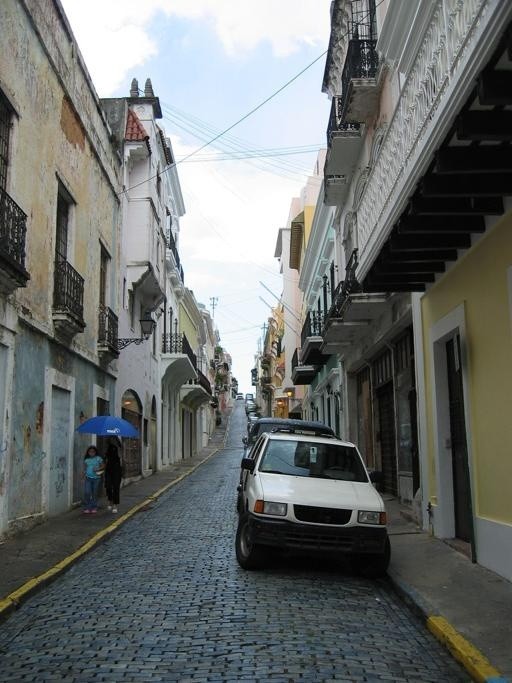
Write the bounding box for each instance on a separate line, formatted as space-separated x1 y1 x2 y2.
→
74 416 140 437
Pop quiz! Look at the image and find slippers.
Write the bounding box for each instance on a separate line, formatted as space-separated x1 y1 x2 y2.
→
105 505 118 514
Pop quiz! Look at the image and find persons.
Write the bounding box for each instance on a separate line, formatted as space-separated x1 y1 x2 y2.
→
103 434 124 513
81 445 104 513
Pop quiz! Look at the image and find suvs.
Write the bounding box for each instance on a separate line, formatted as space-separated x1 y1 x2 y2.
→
235 426 392 577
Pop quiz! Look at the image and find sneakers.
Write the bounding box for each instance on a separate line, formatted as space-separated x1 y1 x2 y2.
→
81 508 99 514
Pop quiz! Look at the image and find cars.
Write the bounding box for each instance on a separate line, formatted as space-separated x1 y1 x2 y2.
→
397 422 415 471
232 390 338 508
